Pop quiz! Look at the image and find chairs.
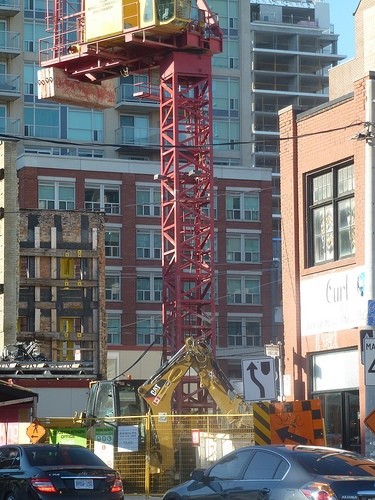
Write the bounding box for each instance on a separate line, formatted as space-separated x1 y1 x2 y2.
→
14 452 58 466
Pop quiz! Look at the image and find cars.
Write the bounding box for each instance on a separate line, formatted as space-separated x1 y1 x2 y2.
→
0 443 124 500
161 444 375 500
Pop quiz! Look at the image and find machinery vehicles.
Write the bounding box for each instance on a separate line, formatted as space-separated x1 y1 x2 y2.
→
25 0 253 497
